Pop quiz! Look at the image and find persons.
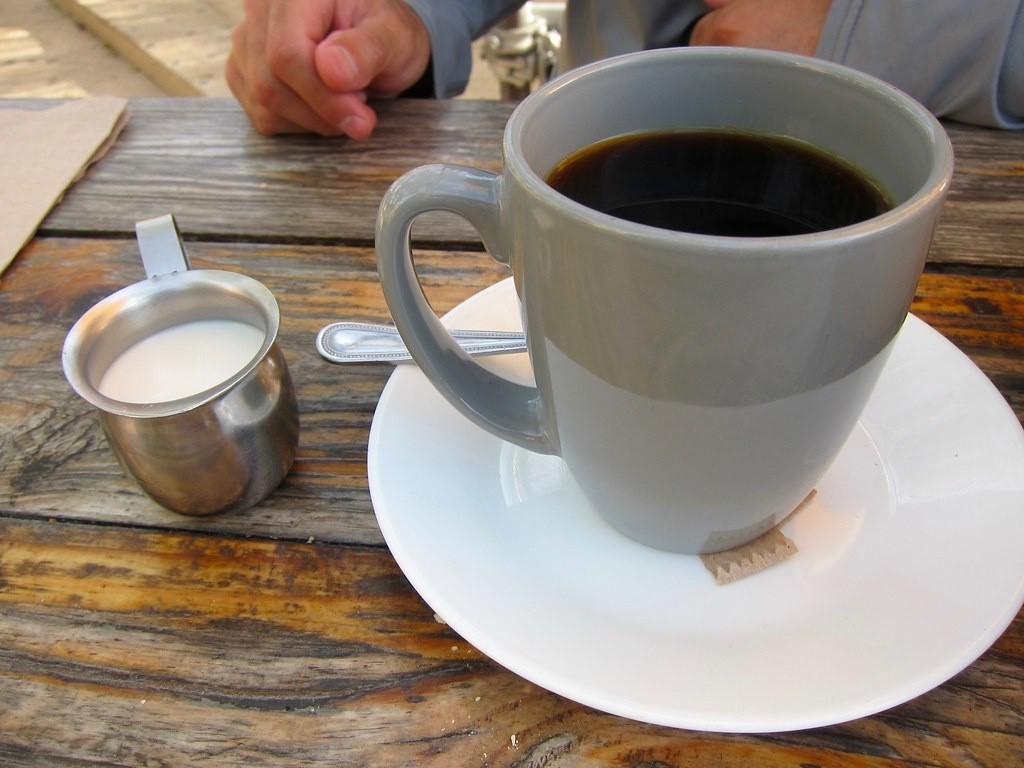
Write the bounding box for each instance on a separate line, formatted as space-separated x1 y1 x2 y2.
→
225 1 1024 142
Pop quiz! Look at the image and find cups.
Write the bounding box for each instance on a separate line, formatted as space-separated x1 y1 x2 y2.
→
373 45 955 553
61 213 301 517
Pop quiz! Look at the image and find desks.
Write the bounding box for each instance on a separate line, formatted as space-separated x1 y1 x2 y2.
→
0 96 1024 768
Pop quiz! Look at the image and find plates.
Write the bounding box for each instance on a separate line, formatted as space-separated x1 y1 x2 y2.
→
366 275 1024 734
